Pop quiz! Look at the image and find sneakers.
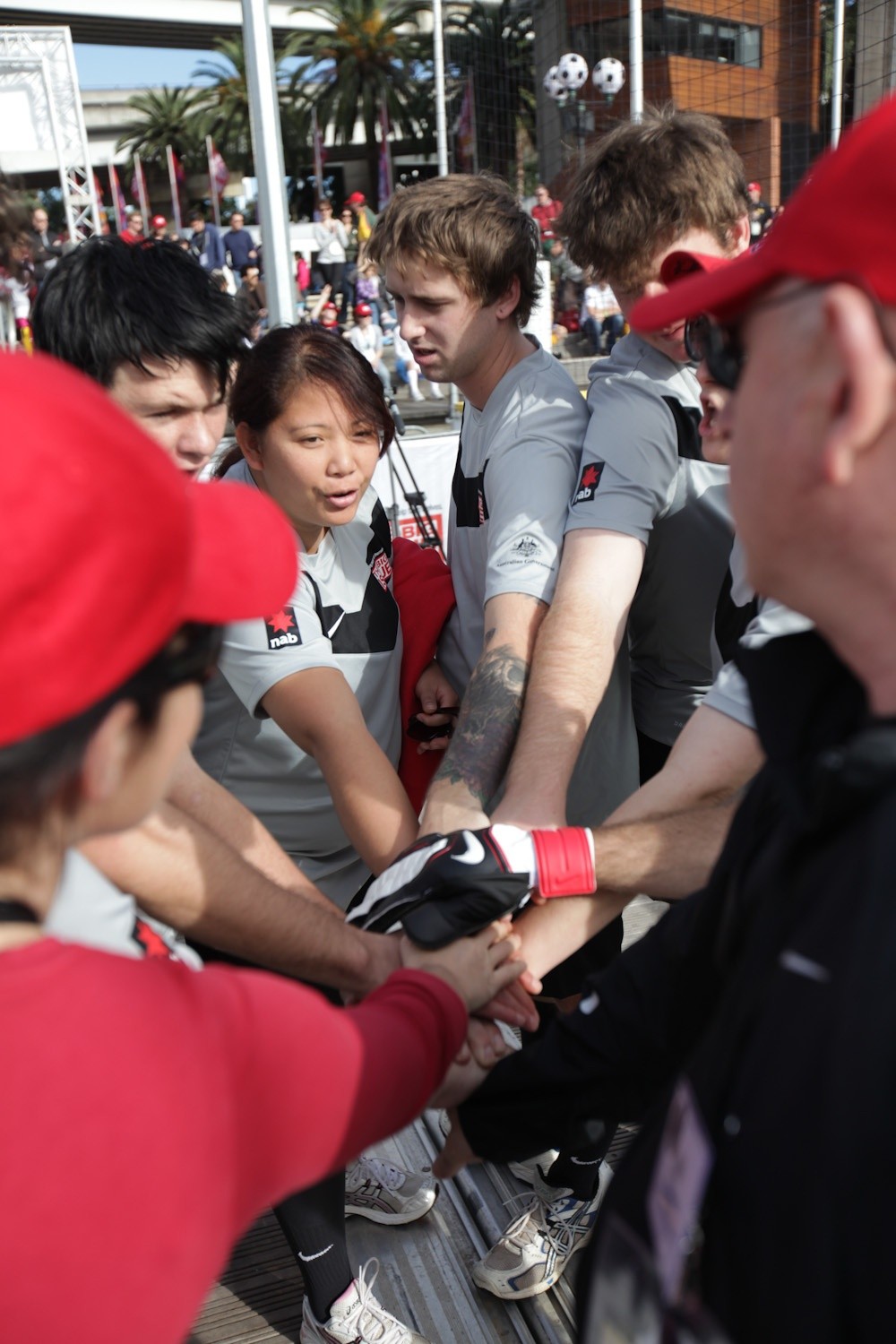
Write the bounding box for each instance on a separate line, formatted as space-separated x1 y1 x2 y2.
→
343 1154 438 1226
300 1256 431 1344
508 1151 560 1185
472 1158 603 1300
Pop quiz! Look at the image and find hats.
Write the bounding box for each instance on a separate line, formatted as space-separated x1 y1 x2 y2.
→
747 181 761 191
654 248 756 325
345 192 365 204
323 302 341 313
151 215 168 230
353 304 371 317
626 88 896 344
1 347 302 749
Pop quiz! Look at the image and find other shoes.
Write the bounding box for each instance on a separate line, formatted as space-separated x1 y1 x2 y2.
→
429 389 445 400
602 349 611 355
409 392 427 401
593 347 602 356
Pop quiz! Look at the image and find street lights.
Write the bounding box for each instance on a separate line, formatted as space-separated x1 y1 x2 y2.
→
542 52 628 170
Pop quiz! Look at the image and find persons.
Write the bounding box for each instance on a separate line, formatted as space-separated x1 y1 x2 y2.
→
428 90 896 1343
0 98 779 1343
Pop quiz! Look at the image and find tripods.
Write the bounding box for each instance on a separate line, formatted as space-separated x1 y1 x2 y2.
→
377 406 448 564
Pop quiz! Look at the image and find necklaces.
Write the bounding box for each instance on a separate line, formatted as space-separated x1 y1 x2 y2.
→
0 897 57 928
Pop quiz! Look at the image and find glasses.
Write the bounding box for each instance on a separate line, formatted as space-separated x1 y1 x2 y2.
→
406 704 460 744
683 273 844 390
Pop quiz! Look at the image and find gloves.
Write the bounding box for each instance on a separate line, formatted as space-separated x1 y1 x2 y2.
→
343 820 596 949
